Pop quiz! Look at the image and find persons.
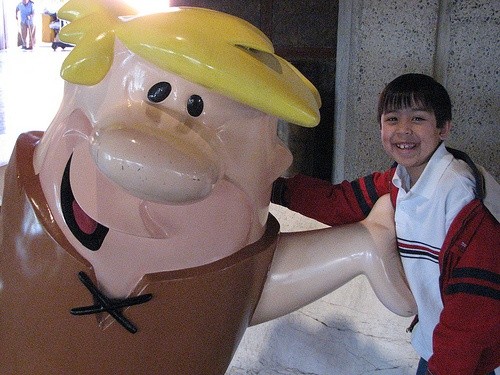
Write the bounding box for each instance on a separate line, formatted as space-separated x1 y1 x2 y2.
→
268 73 499 375
16 0 35 50
